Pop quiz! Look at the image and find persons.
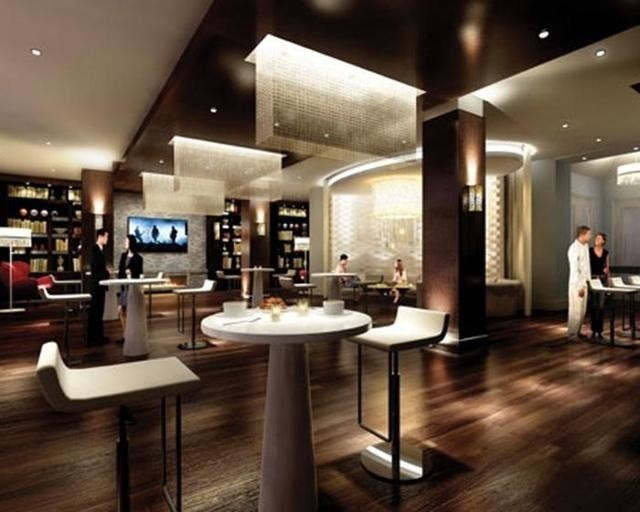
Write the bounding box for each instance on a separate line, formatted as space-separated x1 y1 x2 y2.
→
589 233 610 339
335 255 348 273
134 226 146 243
118 235 142 279
170 226 177 243
392 259 408 285
84 229 109 346
567 225 591 342
151 225 158 241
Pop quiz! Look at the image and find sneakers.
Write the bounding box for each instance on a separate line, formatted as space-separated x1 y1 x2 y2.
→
569 333 607 345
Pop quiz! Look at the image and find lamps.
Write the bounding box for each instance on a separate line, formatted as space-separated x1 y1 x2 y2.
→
617 162 640 186
372 177 424 220
168 135 287 201
139 172 225 217
243 34 426 169
0 226 33 313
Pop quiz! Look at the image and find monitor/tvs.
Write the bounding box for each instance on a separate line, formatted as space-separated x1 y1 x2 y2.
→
127 216 188 253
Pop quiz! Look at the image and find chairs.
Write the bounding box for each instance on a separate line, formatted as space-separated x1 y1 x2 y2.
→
347 305 450 483
36 341 202 511
590 274 640 350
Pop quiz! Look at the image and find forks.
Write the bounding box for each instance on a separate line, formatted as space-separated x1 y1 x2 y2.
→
223 318 261 326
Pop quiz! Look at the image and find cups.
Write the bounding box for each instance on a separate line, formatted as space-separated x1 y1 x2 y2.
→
222 301 248 316
323 300 346 315
140 274 144 279
271 302 281 321
298 298 308 317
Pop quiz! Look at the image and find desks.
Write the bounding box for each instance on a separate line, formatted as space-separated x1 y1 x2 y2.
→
202 305 371 512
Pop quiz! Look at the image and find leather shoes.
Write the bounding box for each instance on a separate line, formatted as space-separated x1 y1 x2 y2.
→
82 336 124 346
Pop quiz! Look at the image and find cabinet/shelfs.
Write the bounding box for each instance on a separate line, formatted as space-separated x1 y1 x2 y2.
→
207 199 241 290
0 176 83 272
268 200 309 285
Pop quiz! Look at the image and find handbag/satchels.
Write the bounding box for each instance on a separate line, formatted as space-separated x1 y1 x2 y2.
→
119 286 127 307
